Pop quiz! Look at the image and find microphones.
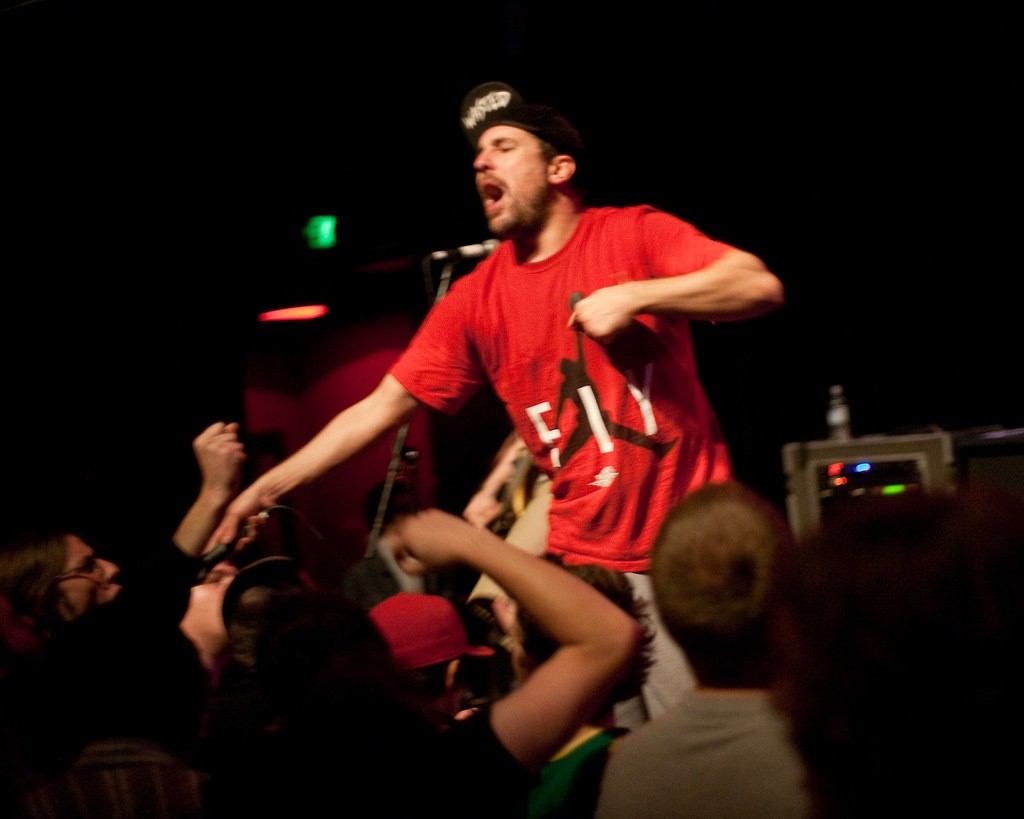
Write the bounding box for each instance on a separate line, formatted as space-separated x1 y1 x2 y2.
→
199 512 270 567
431 239 501 266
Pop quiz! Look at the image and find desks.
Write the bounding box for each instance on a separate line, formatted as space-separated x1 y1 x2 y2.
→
783 430 952 537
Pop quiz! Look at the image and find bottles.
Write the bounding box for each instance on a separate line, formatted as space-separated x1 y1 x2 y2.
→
827 385 852 441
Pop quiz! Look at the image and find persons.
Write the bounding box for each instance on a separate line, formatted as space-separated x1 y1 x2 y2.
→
203 81 786 722
0 420 1024 819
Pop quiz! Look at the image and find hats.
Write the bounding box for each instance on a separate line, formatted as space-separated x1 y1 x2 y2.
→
367 591 495 674
457 82 589 169
